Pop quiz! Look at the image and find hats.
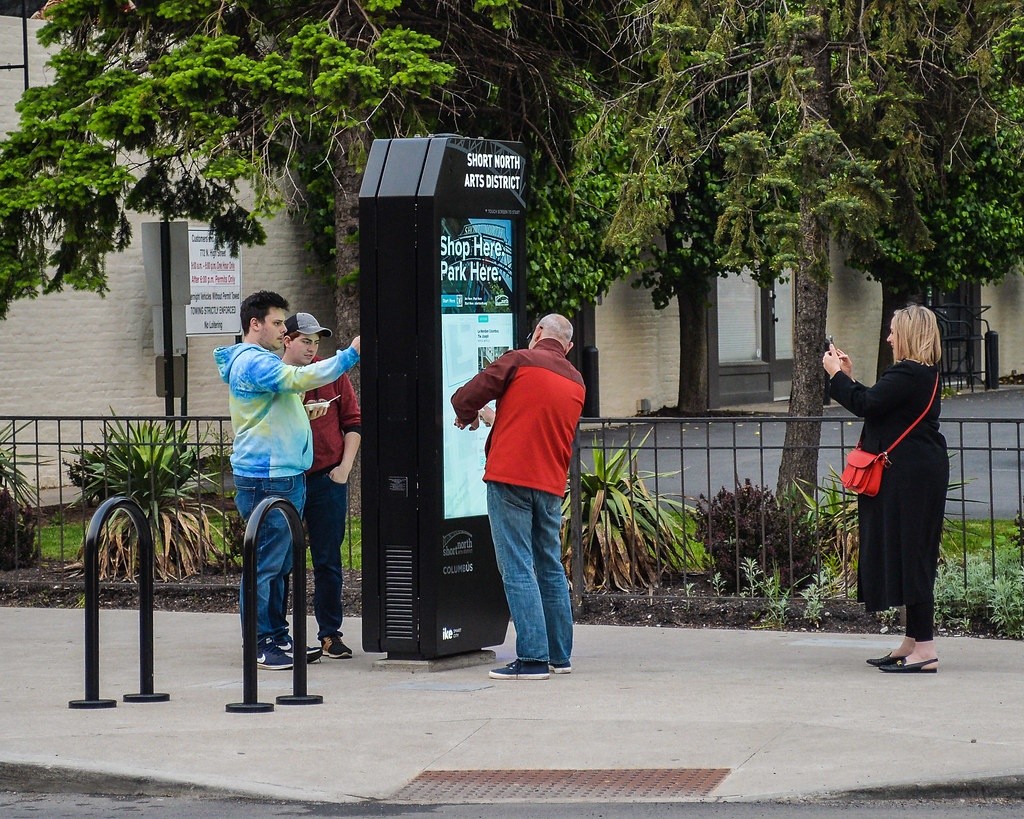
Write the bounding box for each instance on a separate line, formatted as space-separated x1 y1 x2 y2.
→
283 313 333 339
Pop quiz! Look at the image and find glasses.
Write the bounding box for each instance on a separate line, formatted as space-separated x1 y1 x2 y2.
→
902 309 911 319
527 326 543 342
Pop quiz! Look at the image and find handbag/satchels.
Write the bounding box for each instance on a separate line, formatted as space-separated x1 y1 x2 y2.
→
841 448 887 498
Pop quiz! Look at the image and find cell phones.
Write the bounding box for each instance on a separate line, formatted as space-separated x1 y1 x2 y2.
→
829 334 837 353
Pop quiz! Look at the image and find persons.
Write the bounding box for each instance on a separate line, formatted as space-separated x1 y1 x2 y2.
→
822 305 950 673
213 290 360 671
280 313 361 659
450 314 587 679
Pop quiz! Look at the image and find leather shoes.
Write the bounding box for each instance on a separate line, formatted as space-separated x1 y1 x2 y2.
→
865 652 902 666
879 654 939 672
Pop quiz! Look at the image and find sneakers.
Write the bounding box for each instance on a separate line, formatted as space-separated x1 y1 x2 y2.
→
254 639 295 672
488 656 549 679
273 637 322 662
550 660 574 675
320 634 351 658
